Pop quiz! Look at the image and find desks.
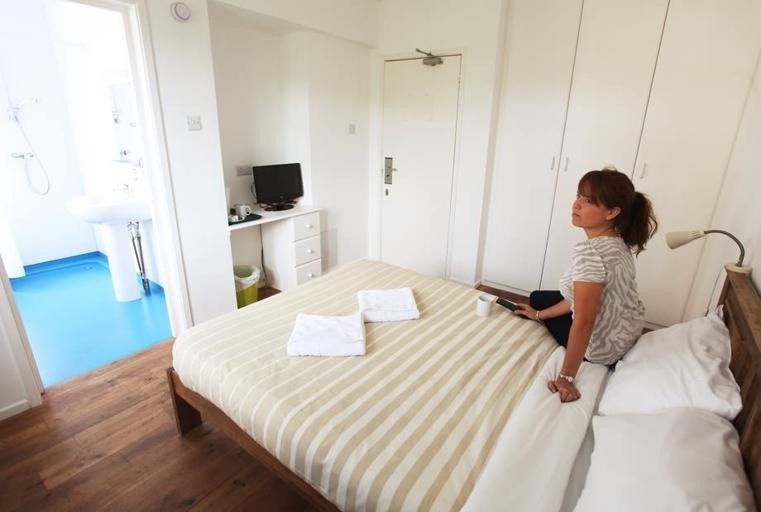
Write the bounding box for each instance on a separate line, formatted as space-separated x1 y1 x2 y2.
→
229 198 325 293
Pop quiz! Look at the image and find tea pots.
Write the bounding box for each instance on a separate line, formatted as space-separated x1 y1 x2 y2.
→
228 202 252 222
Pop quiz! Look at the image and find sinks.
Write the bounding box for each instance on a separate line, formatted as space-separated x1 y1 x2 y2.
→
64 188 153 225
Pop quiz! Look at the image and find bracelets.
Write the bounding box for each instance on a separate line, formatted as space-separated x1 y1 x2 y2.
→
536 310 542 322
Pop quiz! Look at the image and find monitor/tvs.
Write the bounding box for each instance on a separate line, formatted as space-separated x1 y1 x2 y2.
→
252 163 303 211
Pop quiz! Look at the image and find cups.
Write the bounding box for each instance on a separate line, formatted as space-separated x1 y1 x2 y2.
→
476 296 493 316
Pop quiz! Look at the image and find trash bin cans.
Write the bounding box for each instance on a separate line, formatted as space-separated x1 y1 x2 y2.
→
233 265 260 309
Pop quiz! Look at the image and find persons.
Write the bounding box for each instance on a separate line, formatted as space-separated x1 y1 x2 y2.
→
512 167 657 403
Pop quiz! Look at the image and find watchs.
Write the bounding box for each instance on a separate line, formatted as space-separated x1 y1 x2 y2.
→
557 372 577 383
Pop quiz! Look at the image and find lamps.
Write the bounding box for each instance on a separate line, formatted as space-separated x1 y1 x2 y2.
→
665 230 757 278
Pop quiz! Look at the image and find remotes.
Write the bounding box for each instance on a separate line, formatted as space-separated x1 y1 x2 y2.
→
496 297 529 319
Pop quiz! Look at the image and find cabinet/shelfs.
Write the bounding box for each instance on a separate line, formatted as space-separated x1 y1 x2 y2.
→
478 0 668 314
627 2 761 335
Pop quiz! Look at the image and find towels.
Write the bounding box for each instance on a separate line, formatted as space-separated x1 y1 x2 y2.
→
357 287 423 323
287 311 369 359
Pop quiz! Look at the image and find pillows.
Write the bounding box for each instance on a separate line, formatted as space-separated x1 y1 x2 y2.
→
577 404 758 512
597 300 746 417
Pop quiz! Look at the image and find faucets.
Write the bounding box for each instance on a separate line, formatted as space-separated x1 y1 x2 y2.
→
111 181 129 191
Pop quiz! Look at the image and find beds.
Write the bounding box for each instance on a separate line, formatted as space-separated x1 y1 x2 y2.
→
162 249 761 512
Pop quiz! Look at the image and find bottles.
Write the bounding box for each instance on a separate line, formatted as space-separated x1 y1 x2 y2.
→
225 187 231 215
